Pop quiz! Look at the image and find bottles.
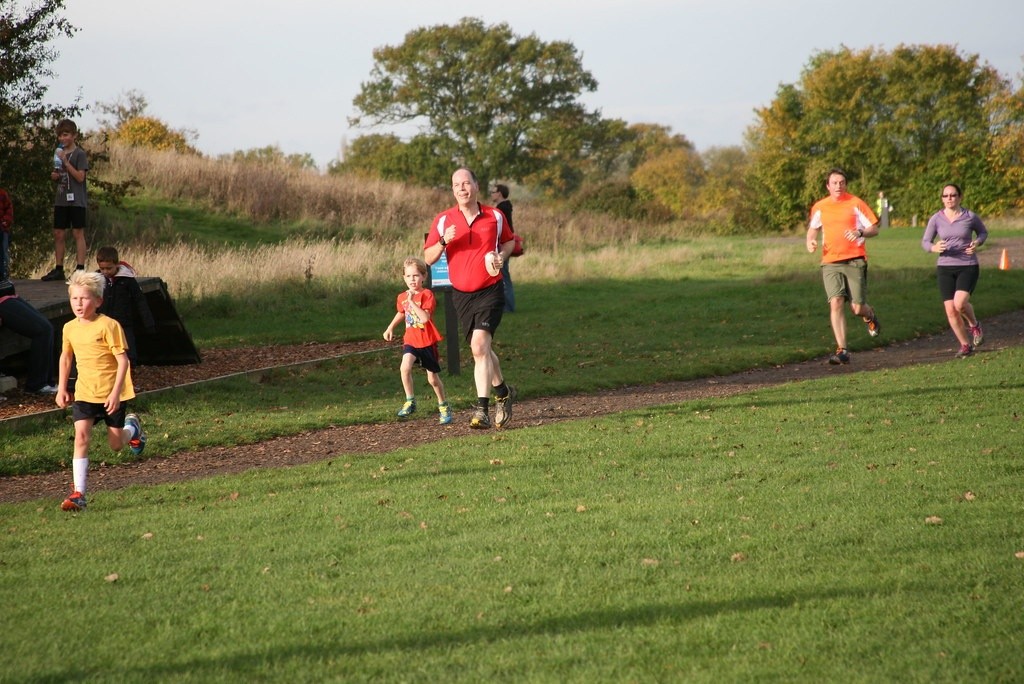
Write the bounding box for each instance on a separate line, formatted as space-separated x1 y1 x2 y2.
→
53 144 63 169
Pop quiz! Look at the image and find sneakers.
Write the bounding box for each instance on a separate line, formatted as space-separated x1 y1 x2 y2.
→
125 413 146 454
970 321 984 346
495 385 513 427
61 491 87 511
470 406 490 429
398 401 416 416
863 306 880 337
438 402 451 424
829 348 850 365
955 343 973 358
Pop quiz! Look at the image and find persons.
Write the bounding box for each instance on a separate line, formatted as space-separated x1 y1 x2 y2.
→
56 270 145 511
383 258 452 425
422 168 515 428
807 169 880 366
877 191 893 228
42 119 89 282
0 187 57 394
94 247 137 287
492 185 515 313
921 184 988 358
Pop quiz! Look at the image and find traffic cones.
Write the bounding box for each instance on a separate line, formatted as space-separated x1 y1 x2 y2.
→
999 248 1009 271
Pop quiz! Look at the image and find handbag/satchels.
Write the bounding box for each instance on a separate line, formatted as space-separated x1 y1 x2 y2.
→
510 234 524 257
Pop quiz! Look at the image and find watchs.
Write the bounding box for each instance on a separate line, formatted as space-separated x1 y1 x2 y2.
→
439 236 447 246
858 229 863 237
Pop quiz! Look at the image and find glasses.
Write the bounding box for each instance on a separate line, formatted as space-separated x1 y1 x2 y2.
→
942 194 959 198
490 190 499 194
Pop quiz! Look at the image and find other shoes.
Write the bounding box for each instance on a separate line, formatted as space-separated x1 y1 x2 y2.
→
41 269 65 281
39 383 60 394
